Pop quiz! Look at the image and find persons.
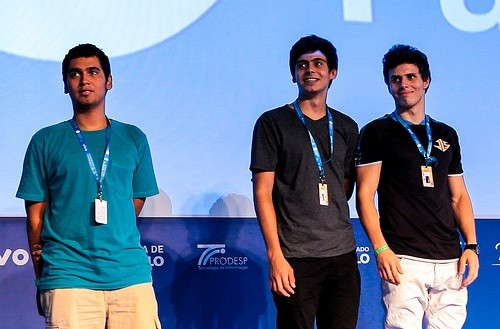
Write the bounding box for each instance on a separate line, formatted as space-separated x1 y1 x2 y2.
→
250 33 361 329
355 41 480 329
16 44 162 329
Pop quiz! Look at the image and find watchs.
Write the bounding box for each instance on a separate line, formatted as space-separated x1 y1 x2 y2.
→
463 243 480 258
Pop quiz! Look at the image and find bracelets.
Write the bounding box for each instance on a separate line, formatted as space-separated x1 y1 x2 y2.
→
374 244 389 255
34 279 41 286
